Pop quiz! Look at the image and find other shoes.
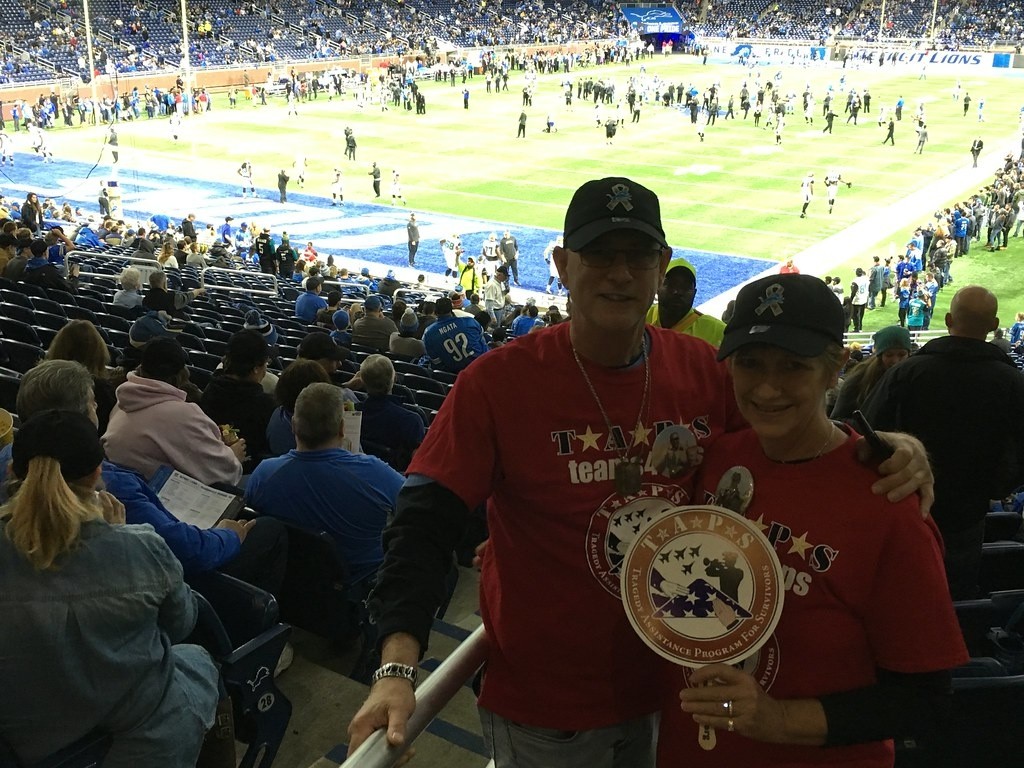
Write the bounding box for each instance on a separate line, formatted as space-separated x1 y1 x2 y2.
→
272 641 293 678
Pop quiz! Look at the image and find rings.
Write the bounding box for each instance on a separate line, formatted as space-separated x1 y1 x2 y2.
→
728 718 735 732
722 700 733 718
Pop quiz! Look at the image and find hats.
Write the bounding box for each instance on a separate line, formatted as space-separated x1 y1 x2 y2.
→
244 309 278 346
872 325 911 360
142 335 185 378
716 274 843 365
497 266 510 277
332 310 348 329
363 296 383 312
564 176 668 254
298 332 350 364
12 409 108 481
400 308 419 332
663 258 697 285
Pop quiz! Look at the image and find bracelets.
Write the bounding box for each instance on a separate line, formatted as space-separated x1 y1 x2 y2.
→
372 663 418 691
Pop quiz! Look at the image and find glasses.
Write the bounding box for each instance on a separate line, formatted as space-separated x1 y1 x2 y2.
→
576 246 666 268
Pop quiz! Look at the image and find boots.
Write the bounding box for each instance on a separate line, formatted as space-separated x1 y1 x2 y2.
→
546 284 552 293
558 290 565 296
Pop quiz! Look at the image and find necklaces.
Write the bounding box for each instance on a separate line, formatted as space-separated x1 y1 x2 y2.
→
772 422 834 463
571 335 649 495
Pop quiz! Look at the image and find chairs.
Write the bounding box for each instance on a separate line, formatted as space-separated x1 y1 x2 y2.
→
0 0 1024 90
0 237 520 768
892 512 1024 768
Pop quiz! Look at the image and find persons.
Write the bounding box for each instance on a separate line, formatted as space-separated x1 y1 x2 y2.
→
655 273 970 768
645 259 727 349
716 472 750 514
824 311 1024 602
0 193 572 768
655 432 691 479
0 0 1024 344
346 177 936 768
